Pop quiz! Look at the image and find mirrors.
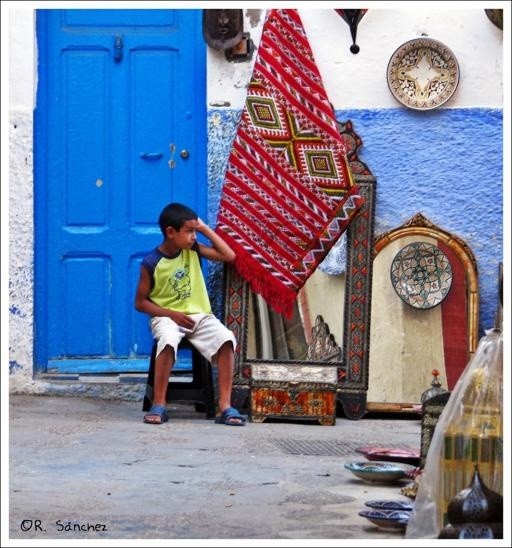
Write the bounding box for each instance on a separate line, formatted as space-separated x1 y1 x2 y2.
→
222 104 376 421
365 211 479 414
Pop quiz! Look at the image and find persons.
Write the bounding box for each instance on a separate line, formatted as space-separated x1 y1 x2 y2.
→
134 202 246 426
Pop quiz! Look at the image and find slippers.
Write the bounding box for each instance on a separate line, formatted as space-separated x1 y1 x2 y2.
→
215 407 247 425
143 404 168 423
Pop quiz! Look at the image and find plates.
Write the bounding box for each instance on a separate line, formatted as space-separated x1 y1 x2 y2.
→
386 37 460 110
347 447 420 532
389 241 453 311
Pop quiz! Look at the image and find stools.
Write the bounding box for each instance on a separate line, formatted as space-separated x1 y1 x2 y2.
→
143 337 216 419
